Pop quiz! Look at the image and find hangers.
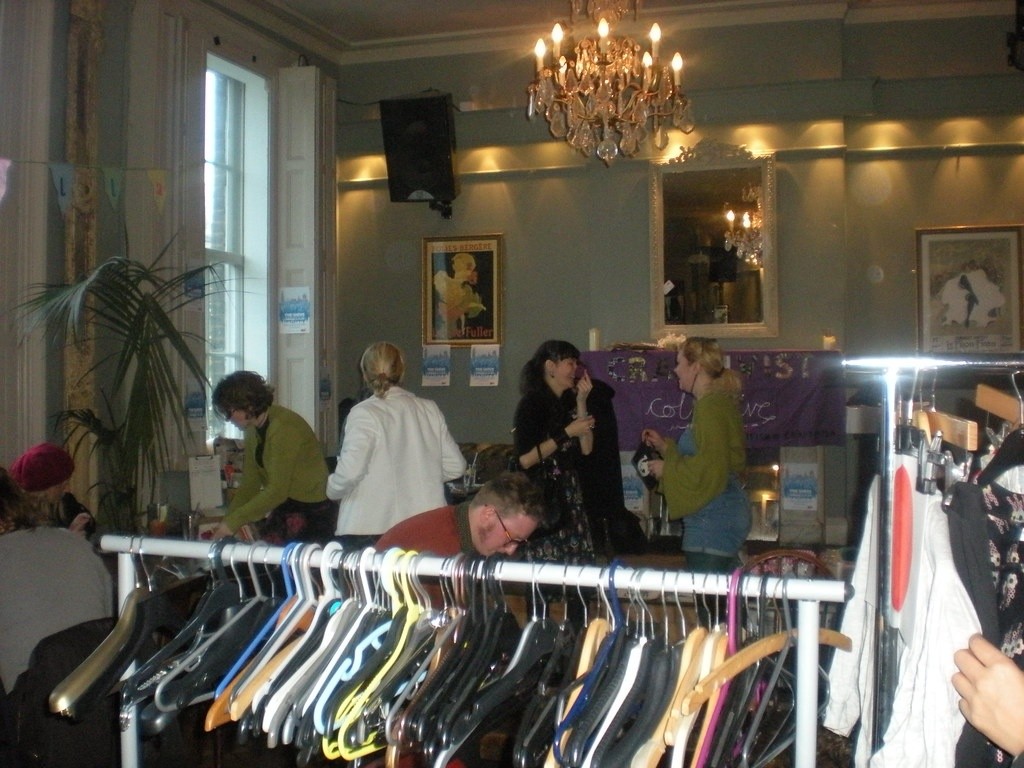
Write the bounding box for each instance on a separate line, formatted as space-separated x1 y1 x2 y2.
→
48 535 831 768
895 358 1023 452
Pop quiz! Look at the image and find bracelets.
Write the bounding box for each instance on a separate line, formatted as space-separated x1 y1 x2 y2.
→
552 428 570 448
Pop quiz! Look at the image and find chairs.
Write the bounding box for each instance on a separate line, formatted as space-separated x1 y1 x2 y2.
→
738 550 842 632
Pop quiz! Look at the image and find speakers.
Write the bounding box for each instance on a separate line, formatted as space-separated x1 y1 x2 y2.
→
379 90 462 202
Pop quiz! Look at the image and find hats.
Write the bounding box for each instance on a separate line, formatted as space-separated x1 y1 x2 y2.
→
11 445 75 492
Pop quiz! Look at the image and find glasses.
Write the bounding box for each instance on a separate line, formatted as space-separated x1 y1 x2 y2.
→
485 503 528 546
222 408 240 421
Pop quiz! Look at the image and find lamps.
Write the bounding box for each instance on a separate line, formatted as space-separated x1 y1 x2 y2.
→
525 0 695 169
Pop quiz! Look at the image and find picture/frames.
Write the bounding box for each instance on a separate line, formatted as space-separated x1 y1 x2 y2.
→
915 225 1024 354
648 151 780 339
421 233 504 346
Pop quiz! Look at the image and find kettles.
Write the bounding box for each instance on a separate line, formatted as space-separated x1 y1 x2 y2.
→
650 491 683 553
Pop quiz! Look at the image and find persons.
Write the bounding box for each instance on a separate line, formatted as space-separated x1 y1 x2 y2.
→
641 337 752 629
210 370 334 547
508 340 594 633
325 342 465 549
374 471 544 611
0 443 113 698
951 633 1024 768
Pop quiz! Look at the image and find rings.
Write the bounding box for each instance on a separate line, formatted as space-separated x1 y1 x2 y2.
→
589 424 594 430
652 472 655 476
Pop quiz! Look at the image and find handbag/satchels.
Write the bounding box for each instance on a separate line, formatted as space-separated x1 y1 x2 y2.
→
632 441 658 490
530 445 561 531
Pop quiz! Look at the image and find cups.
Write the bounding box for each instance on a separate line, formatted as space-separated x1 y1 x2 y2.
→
588 328 601 352
226 488 237 507
148 502 168 537
823 336 835 350
463 464 476 487
181 512 199 542
